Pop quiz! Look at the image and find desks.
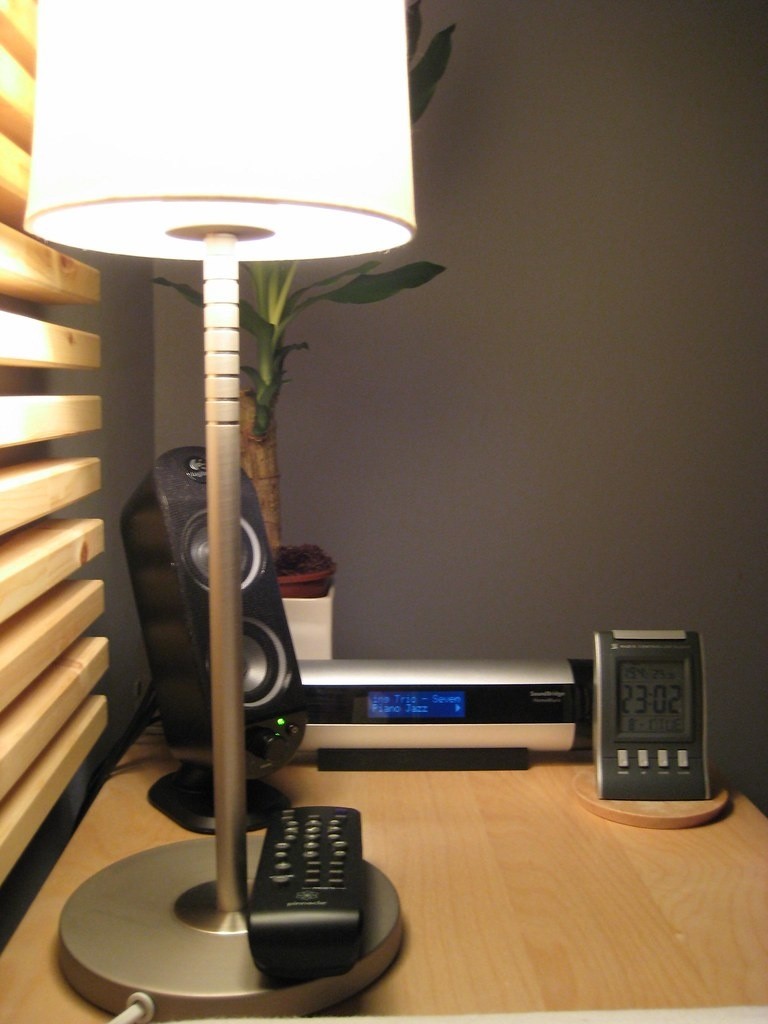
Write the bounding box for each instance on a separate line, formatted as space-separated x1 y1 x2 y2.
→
1 713 768 1024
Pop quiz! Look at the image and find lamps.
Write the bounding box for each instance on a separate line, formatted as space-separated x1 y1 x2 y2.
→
22 0 416 1019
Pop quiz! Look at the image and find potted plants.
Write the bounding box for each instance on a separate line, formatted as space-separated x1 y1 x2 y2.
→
155 0 458 598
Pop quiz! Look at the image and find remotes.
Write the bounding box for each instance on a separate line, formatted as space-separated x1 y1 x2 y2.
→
247 805 363 977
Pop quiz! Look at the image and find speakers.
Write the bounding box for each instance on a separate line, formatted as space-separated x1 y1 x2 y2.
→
121 446 305 835
